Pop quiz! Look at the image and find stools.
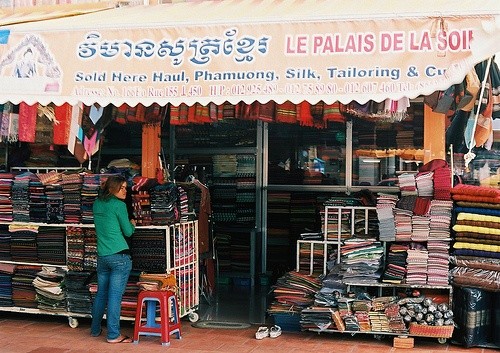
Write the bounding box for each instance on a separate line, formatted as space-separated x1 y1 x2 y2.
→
132 290 182 347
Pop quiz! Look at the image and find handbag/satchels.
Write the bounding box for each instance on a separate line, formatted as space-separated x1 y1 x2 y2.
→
137 272 177 291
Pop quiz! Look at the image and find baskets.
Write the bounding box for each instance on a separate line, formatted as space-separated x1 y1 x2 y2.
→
410 323 453 337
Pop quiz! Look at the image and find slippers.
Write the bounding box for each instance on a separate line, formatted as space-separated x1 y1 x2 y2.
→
117 335 133 343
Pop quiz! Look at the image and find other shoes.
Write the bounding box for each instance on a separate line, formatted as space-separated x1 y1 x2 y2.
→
270 325 281 338
256 326 268 339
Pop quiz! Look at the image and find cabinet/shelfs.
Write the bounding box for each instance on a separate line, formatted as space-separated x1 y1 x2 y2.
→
309 238 454 345
0 220 200 328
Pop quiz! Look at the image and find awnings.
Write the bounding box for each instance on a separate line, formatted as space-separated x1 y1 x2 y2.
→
0 0 500 108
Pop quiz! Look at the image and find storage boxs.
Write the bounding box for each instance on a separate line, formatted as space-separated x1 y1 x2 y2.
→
271 314 301 333
408 321 454 338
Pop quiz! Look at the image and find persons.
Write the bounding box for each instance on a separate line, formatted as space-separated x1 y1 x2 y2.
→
89 175 136 344
